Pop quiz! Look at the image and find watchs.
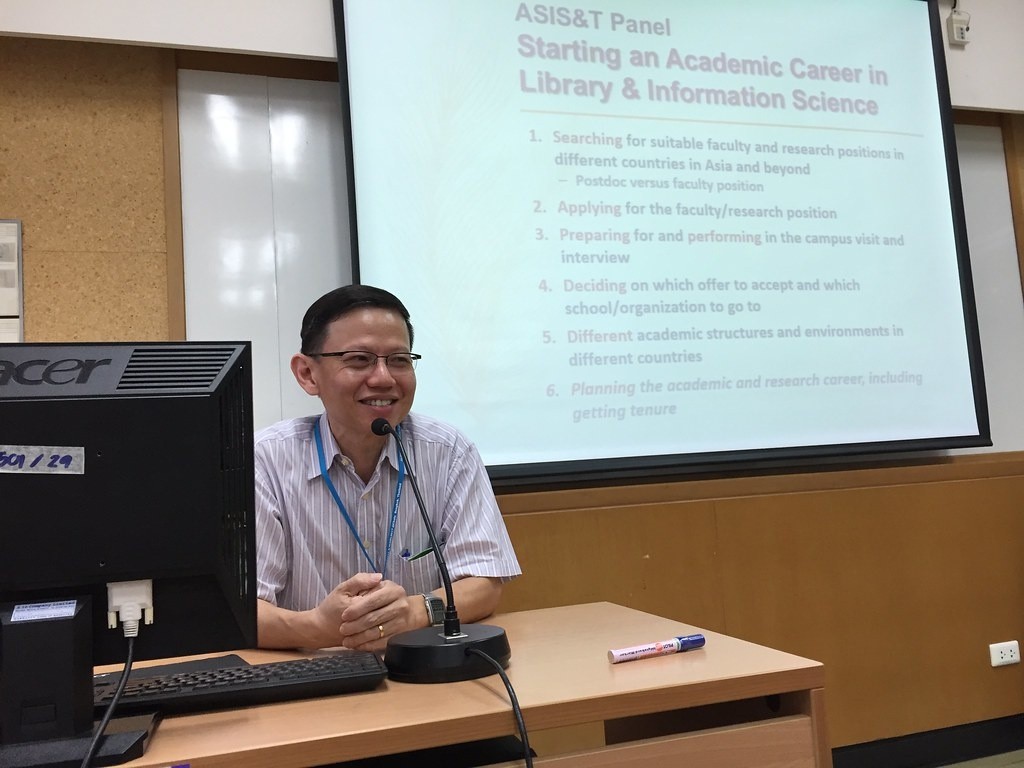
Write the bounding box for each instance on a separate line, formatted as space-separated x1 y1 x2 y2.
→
418 590 447 625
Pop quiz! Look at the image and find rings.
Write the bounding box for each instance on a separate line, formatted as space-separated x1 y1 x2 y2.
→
378 626 385 638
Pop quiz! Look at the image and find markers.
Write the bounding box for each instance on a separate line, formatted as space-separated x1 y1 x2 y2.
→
608 633 707 664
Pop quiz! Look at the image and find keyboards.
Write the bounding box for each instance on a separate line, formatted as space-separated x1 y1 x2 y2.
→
92 650 388 720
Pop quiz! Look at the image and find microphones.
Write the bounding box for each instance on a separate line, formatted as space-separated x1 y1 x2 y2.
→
371 418 512 684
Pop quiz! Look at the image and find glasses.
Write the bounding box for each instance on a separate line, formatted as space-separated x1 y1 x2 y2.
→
309 351 421 371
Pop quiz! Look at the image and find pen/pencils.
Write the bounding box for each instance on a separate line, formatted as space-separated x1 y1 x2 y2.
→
402 547 411 560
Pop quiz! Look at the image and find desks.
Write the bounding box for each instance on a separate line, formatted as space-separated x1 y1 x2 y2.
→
93 600 834 768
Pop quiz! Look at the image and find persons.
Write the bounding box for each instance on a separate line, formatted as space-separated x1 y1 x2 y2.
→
252 283 523 652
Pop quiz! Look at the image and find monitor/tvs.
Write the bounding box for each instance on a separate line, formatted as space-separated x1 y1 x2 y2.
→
0 341 256 768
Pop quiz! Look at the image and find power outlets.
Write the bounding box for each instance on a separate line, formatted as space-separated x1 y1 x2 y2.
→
989 640 1021 667
